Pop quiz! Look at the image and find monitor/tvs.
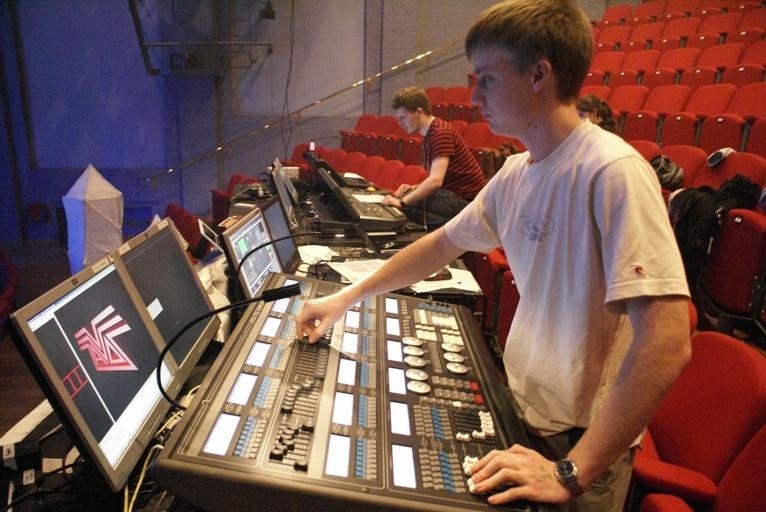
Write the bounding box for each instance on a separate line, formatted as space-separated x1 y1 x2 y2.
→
197 218 224 254
117 215 225 379
261 193 301 275
272 156 299 205
270 167 297 224
219 207 284 310
5 251 184 493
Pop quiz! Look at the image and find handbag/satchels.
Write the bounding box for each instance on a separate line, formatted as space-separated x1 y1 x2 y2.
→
649 156 685 189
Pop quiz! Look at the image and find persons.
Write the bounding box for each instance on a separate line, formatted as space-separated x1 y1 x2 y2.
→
381 87 487 231
294 0 693 511
575 94 616 132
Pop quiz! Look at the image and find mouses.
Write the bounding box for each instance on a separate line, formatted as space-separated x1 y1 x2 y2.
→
425 268 452 282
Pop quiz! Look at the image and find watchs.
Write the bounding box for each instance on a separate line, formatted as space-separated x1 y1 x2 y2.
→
399 197 408 210
555 458 585 498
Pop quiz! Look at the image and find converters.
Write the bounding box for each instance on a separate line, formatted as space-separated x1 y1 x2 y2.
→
1 438 45 479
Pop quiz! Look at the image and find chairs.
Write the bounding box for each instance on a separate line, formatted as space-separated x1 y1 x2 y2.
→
0 247 18 341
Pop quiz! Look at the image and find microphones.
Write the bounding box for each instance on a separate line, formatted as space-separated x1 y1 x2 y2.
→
304 135 350 153
317 136 339 159
233 229 364 316
156 282 300 409
